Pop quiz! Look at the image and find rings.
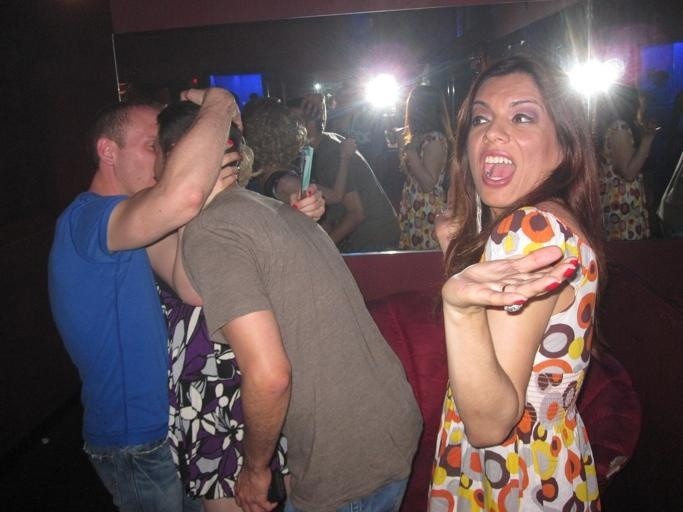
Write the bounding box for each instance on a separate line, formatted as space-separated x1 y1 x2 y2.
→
502 283 524 313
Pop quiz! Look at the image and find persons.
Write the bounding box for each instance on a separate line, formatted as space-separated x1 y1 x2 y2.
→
426 55 611 510
47 87 244 510
155 101 423 510
241 64 661 253
142 138 326 511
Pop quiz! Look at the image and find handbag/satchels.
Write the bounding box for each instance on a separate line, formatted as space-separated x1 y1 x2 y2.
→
576 348 641 487
